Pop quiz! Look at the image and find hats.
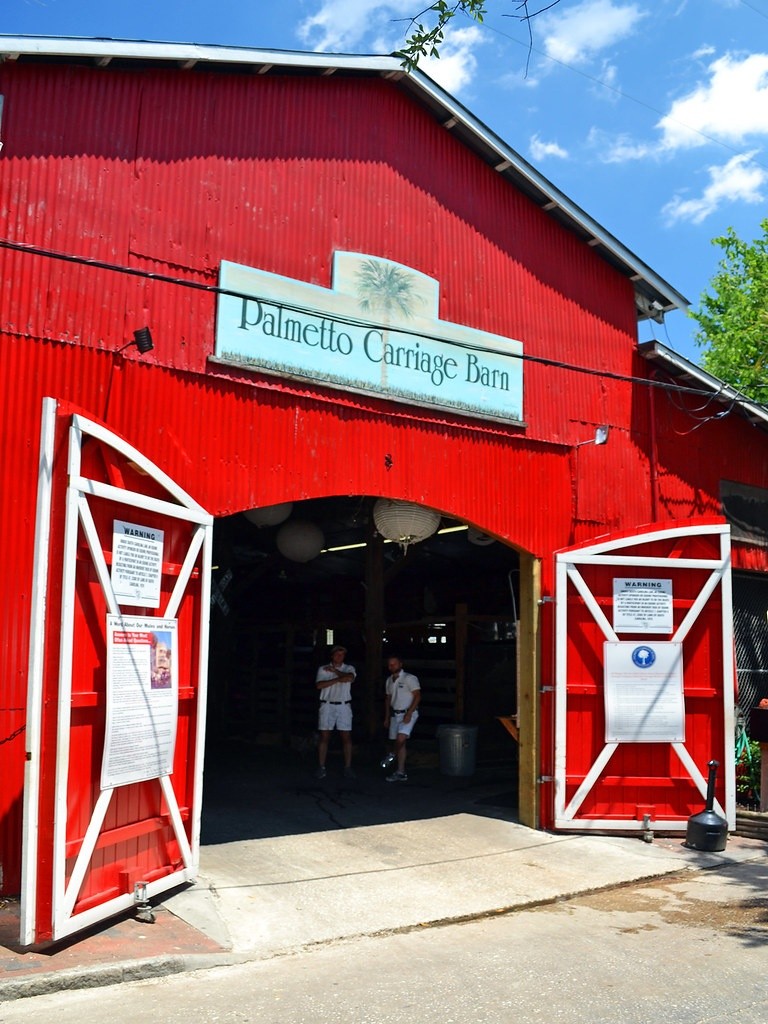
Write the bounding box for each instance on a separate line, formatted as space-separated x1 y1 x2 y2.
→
330 645 346 652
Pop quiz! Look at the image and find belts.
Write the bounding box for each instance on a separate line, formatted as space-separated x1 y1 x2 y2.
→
321 699 351 706
395 709 415 714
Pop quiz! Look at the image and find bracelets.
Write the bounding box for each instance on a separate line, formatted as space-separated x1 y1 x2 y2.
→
333 668 338 672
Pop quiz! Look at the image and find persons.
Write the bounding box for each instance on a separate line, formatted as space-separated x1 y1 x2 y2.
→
382 657 421 782
316 646 356 779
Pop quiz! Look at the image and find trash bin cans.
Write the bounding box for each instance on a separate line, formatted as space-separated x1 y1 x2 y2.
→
437 723 479 781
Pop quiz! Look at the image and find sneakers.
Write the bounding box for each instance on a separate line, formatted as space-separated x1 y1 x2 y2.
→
343 765 358 780
316 766 326 780
386 770 408 783
380 753 396 769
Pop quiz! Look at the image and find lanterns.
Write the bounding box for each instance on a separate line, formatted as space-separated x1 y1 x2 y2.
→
468 527 496 545
245 502 292 526
278 521 324 562
374 497 440 555
